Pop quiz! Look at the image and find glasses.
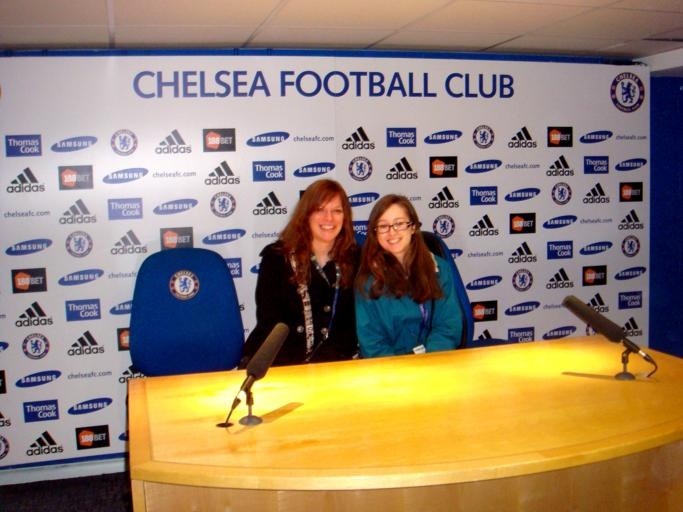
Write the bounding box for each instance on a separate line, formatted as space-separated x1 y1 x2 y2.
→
373 221 415 234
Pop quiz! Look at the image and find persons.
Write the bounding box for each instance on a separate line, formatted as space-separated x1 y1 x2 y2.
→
353 195 463 360
237 179 363 365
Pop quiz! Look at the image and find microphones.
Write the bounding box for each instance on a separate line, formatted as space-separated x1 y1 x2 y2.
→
561 293 657 365
232 322 290 408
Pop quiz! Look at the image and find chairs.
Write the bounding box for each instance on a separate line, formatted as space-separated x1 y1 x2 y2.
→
130 245 244 372
421 228 509 348
